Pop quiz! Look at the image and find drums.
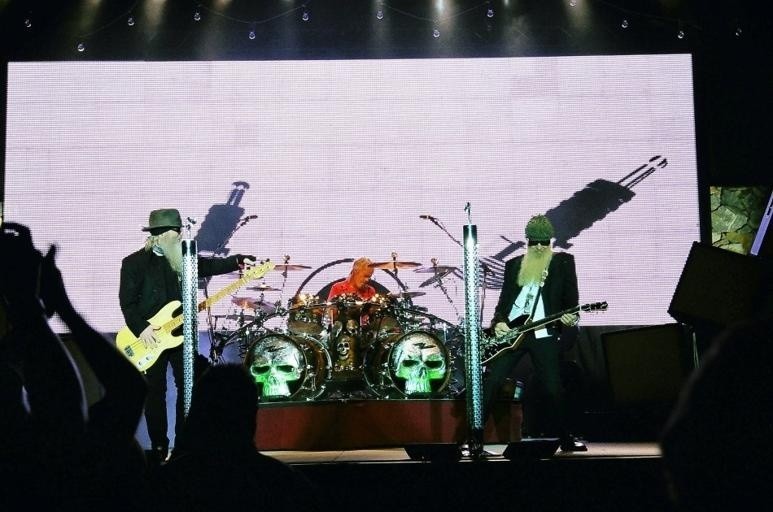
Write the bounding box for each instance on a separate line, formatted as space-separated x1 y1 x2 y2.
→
362 330 452 398
287 307 328 337
246 335 330 402
368 307 403 339
329 303 361 334
328 331 361 382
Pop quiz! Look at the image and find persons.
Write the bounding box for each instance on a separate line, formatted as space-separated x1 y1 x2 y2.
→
483 215 588 451
1 223 153 512
151 363 295 512
119 208 255 456
327 257 377 328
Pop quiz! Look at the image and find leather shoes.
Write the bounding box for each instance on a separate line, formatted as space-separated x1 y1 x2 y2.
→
566 441 588 451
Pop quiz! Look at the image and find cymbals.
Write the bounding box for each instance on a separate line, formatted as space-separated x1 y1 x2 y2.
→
387 292 426 299
418 272 450 287
367 261 421 269
271 263 311 272
244 283 281 291
414 265 456 273
232 297 276 311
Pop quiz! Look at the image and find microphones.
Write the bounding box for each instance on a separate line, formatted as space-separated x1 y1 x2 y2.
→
392 253 397 269
419 215 434 221
282 254 290 279
431 258 438 276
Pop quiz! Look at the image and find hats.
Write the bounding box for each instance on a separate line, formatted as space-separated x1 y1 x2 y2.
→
141 209 184 231
525 214 554 240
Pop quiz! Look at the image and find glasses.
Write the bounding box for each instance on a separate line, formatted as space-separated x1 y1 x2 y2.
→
528 239 551 246
170 226 180 232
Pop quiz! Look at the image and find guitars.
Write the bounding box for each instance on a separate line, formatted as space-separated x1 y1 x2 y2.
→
116 258 275 372
478 302 608 365
